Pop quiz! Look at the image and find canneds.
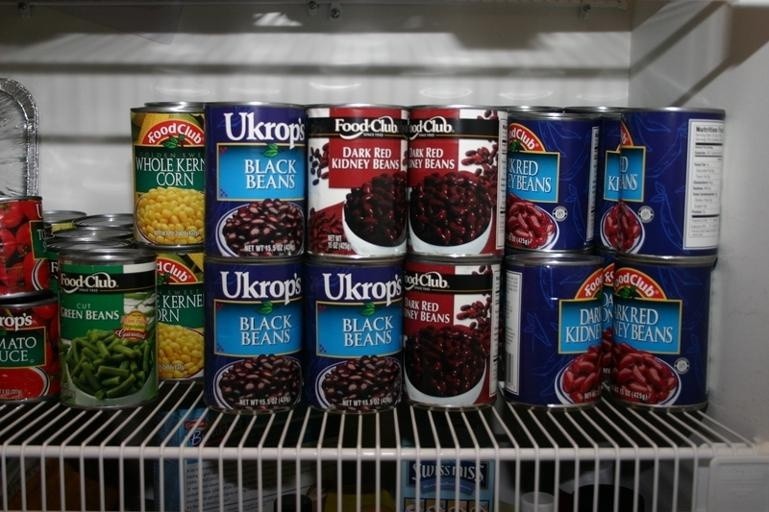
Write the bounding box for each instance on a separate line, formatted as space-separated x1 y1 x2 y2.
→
0 99 726 415
